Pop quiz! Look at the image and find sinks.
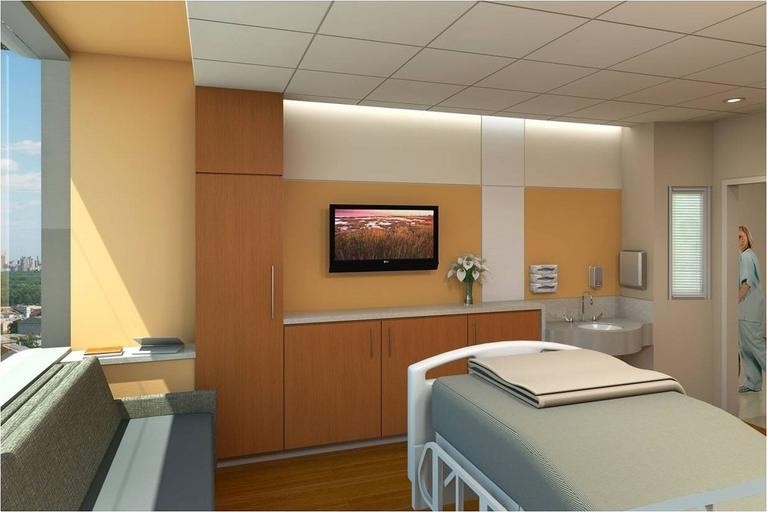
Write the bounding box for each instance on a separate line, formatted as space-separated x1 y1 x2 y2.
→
578 323 624 331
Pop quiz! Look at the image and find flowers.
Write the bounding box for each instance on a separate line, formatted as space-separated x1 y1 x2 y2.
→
446 252 491 303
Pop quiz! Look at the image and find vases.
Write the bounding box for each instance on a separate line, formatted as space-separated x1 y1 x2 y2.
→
464 279 474 306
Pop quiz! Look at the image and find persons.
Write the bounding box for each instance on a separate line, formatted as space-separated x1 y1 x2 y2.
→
738 225 765 394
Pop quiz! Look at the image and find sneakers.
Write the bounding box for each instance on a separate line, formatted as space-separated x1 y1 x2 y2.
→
739 385 753 393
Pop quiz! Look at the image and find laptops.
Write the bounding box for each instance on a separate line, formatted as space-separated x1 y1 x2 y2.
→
132 337 184 354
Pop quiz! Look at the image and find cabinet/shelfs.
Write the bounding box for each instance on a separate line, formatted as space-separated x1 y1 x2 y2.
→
195 173 284 461
285 311 541 450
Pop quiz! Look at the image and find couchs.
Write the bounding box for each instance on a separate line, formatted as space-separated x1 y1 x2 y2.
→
1 356 218 511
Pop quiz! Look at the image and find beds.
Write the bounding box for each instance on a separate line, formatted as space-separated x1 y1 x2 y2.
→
407 340 765 511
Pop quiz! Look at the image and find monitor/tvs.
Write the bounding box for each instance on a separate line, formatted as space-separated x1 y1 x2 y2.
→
327 203 438 274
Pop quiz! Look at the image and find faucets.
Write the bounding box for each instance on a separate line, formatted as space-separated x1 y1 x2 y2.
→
581 290 593 321
